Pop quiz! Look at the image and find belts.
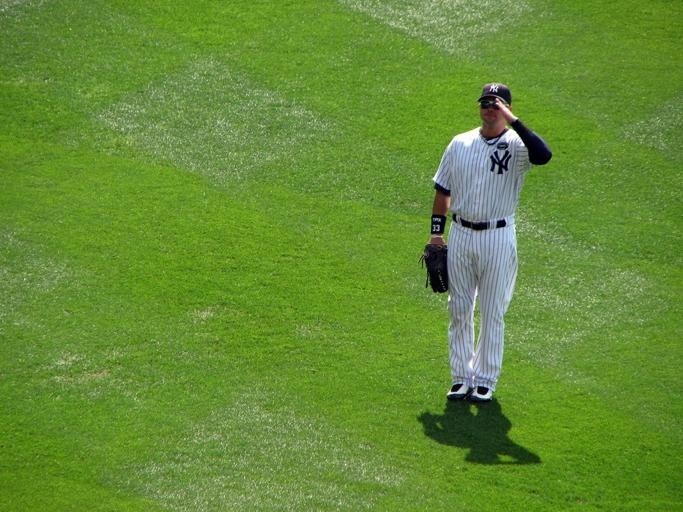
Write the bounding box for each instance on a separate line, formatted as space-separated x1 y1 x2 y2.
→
452 213 507 231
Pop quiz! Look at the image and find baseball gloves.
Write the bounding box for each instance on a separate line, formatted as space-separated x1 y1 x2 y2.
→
423 244 447 293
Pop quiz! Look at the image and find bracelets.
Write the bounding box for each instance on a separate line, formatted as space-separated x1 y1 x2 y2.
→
431 215 447 239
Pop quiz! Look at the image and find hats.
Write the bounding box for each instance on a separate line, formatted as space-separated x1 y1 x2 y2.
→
478 83 512 107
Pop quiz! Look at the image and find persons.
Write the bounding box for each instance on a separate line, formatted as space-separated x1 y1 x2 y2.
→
429 82 552 404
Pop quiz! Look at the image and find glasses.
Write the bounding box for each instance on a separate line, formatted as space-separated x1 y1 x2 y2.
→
478 100 509 110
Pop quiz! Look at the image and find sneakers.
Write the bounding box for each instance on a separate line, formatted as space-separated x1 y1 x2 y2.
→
465 385 493 402
446 384 473 400
